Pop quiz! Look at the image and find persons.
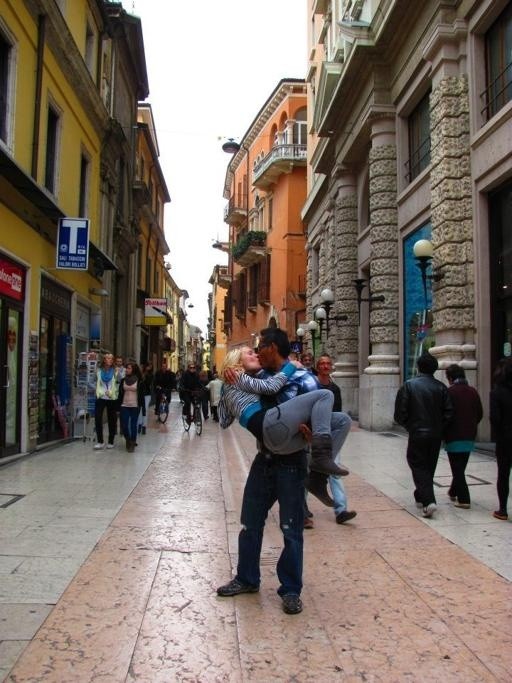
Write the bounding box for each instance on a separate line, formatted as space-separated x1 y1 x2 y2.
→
89 351 223 454
394 351 512 521
215 322 357 614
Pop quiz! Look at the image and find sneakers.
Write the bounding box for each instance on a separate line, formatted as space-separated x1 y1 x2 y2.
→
420 503 436 517
280 594 302 614
305 501 313 517
336 510 356 523
93 441 105 449
217 579 259 596
455 500 470 508
303 518 312 528
107 443 113 448
493 510 508 520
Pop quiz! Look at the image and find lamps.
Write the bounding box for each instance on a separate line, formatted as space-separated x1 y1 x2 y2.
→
223 138 240 153
321 288 347 339
413 239 443 306
297 328 308 354
89 288 109 296
307 320 320 368
316 308 330 331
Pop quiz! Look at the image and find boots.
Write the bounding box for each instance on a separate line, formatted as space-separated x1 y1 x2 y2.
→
138 425 146 434
311 433 348 476
304 472 337 507
125 438 137 452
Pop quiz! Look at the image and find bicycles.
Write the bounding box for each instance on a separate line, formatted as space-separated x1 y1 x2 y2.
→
177 386 204 436
155 386 175 423
